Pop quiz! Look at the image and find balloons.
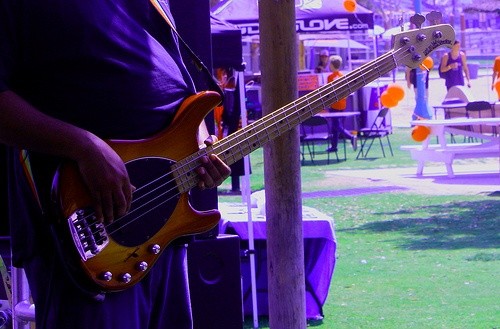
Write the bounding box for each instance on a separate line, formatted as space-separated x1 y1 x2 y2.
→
421 56 433 70
380 90 398 108
344 0 357 13
411 126 430 142
387 86 404 100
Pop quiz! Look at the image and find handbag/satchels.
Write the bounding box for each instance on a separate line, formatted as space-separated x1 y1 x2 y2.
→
438 52 451 79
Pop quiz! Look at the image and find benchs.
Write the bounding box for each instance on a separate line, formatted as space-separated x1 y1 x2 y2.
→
399 143 500 163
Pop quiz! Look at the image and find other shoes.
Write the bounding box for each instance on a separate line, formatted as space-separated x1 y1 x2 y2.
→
325 147 337 153
351 135 358 151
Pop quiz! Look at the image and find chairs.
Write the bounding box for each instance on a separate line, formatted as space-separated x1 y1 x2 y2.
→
300 116 338 166
441 98 497 143
355 107 394 160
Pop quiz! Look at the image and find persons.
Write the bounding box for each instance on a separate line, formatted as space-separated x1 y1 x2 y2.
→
439 40 471 92
491 56 500 101
314 50 330 72
326 56 357 152
0 0 232 329
405 65 432 124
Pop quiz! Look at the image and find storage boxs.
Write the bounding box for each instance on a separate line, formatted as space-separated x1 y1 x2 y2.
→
297 73 325 91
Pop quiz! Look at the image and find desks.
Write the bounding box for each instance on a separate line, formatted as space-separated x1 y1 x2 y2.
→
220 84 389 143
432 102 499 144
410 118 500 178
217 201 337 323
311 111 361 161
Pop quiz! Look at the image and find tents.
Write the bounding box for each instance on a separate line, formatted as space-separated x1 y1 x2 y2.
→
209 0 382 111
210 12 258 329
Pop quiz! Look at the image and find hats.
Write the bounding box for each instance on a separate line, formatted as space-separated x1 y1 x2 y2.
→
319 49 330 57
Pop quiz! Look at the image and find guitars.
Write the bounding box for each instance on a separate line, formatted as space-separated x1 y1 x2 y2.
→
50 10 456 295
